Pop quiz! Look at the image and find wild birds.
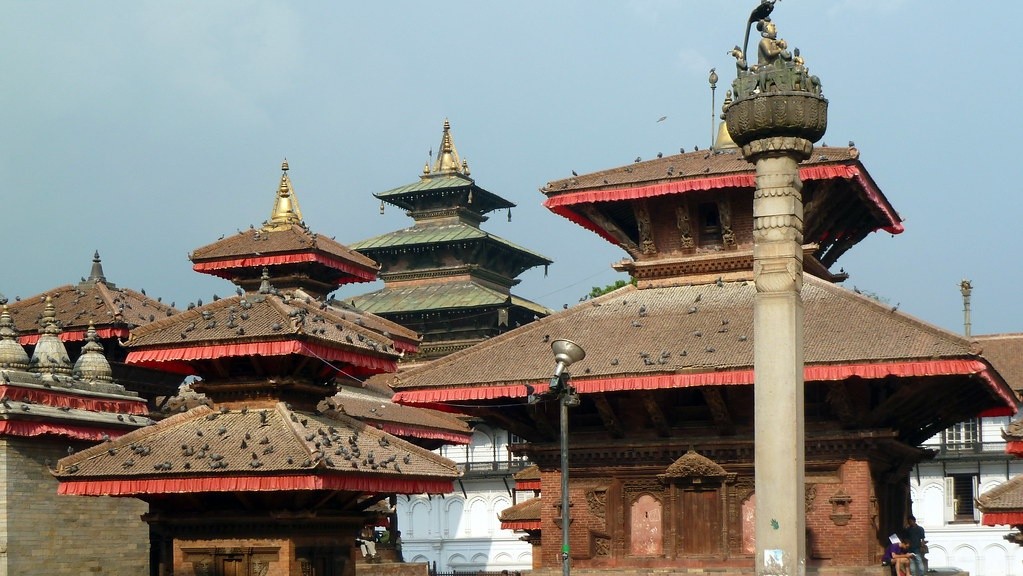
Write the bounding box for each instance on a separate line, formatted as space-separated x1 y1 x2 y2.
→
40 399 415 475
144 282 395 354
212 216 337 250
542 143 746 191
0 352 144 423
838 263 903 315
514 274 752 374
817 139 857 161
0 275 174 334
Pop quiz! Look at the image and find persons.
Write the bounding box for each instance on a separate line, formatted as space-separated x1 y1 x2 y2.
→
726 16 825 98
354 521 391 561
899 515 926 576
881 533 917 576
